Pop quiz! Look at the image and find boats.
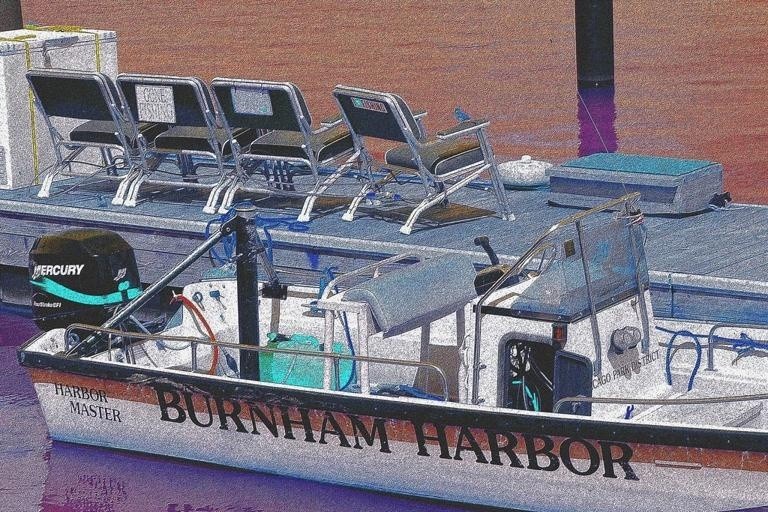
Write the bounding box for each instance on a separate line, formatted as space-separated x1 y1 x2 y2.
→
17 192 768 512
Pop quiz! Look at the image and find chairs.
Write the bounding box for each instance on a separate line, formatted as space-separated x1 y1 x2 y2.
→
210 76 381 223
115 73 281 215
26 67 189 206
332 84 516 235
